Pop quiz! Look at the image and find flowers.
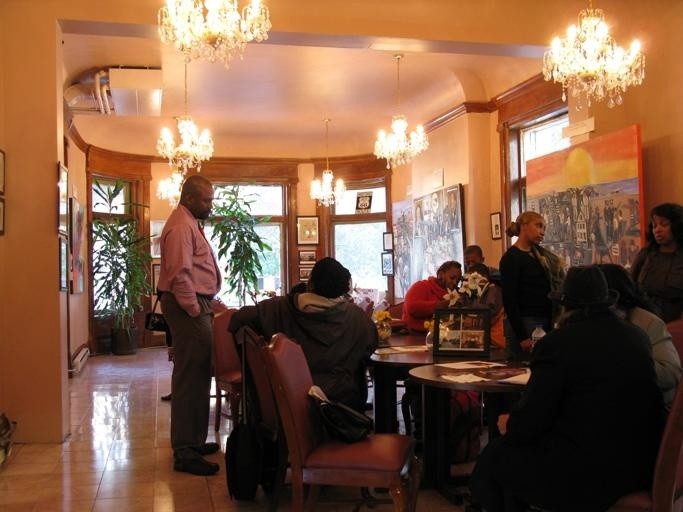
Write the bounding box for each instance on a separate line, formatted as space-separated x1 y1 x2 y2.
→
374 310 392 337
443 272 490 307
424 319 449 340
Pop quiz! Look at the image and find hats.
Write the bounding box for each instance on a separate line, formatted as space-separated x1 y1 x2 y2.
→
557 262 616 308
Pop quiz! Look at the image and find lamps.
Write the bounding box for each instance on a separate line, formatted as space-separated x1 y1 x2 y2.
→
156 0 272 70
310 118 347 207
156 61 213 175
374 53 429 169
156 166 185 209
542 0 648 111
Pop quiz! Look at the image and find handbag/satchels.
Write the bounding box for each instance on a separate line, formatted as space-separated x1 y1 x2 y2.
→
144 296 169 332
312 388 375 444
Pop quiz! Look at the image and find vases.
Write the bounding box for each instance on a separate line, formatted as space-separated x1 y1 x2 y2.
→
375 322 392 342
436 303 492 357
426 332 442 353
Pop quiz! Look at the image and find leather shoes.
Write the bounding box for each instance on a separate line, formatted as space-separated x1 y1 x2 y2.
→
161 392 172 403
174 453 220 477
199 441 221 457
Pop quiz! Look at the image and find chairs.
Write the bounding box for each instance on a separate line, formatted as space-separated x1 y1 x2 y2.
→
386 302 404 321
263 333 424 511
235 326 292 512
213 304 227 313
610 369 683 512
669 318 683 367
213 309 244 433
357 298 373 319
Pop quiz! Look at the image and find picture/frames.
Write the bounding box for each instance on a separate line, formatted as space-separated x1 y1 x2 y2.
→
73 199 87 298
58 161 69 237
383 232 394 250
381 253 394 277
296 216 320 245
299 250 316 265
490 212 503 240
152 263 163 295
391 199 411 299
59 236 70 292
299 267 313 281
432 168 444 188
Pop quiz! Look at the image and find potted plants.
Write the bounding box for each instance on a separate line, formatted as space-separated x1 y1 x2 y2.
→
88 177 158 355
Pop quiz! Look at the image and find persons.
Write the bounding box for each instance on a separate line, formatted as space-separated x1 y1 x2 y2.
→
428 193 438 226
228 283 305 342
464 265 505 348
157 286 173 402
156 175 221 476
463 265 666 512
444 192 459 229
413 203 425 236
402 261 460 333
275 256 378 433
498 211 565 361
462 245 499 273
597 262 681 412
629 203 683 324
